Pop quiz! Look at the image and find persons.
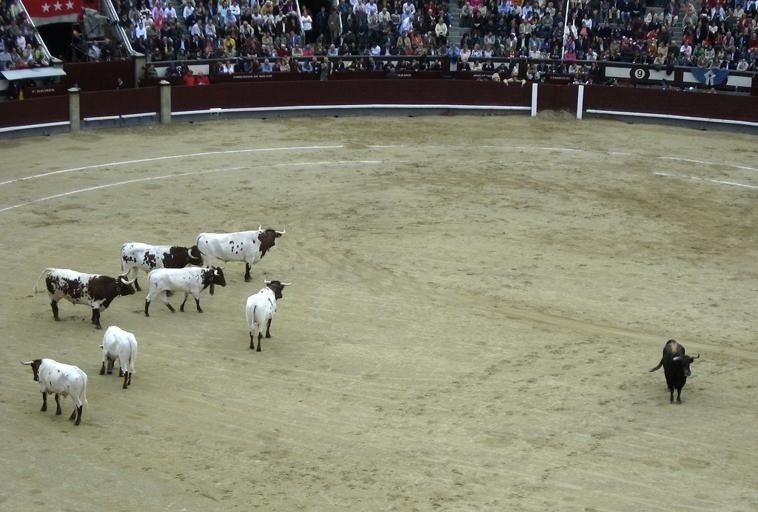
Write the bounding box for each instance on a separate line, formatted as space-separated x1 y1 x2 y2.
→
0 1 756 102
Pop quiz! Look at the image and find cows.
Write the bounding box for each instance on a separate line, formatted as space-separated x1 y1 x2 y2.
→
196 225 285 281
244 279 292 352
33 267 138 328
119 242 208 292
649 337 700 404
97 325 139 388
144 266 227 317
20 358 89 426
